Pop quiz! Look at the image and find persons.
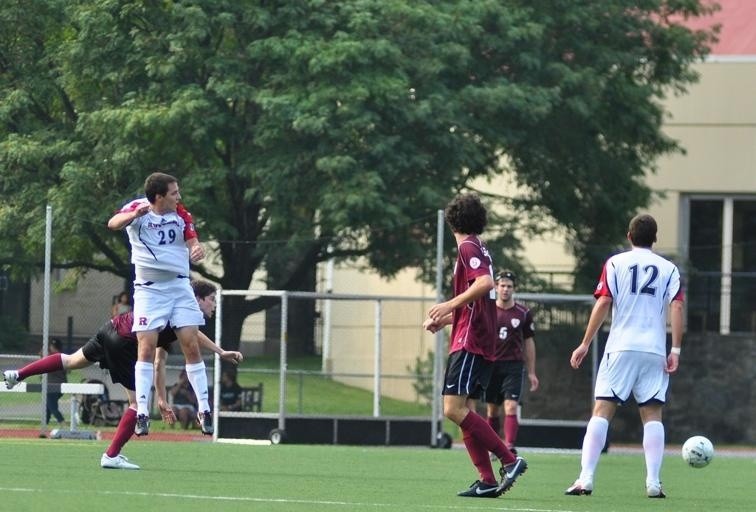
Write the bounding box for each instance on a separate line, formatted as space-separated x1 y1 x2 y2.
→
219 364 243 412
107 173 215 436
112 292 132 318
169 370 198 430
422 192 527 498
3 280 244 469
563 215 685 498
39 338 67 438
481 270 540 460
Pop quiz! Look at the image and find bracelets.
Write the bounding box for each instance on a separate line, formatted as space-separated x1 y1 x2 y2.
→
670 347 681 355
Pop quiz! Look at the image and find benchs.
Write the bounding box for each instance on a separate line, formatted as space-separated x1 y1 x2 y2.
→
149 382 262 419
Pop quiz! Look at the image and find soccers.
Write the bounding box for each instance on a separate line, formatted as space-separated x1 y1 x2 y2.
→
682 436 714 468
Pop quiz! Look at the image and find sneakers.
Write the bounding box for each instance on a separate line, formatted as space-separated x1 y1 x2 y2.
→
456 479 500 498
134 413 150 436
196 410 214 435
3 370 19 389
645 480 666 498
488 447 528 497
100 452 140 469
565 479 593 495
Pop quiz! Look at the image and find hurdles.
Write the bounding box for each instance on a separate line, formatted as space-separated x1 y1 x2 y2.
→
0 382 105 440
